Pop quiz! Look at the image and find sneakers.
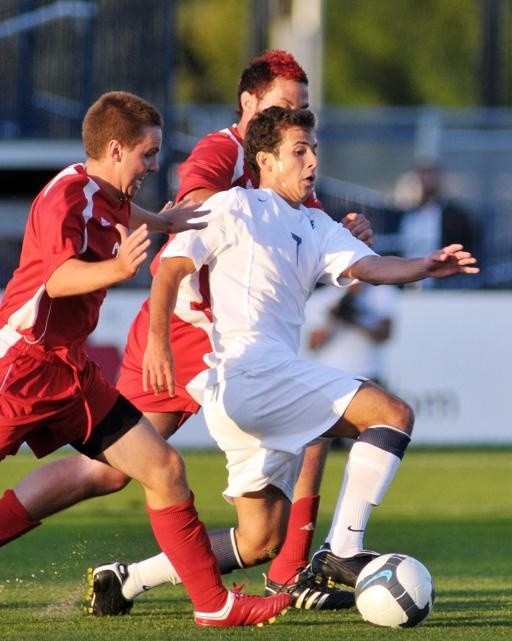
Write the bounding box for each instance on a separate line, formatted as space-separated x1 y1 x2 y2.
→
308 542 382 594
263 566 359 611
81 561 138 617
190 586 297 628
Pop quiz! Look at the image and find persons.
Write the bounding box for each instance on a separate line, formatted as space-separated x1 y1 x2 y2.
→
388 162 482 291
2 46 379 595
2 90 295 630
77 106 484 617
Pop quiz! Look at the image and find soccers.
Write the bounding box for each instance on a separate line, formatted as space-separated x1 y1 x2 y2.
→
354 552 436 629
